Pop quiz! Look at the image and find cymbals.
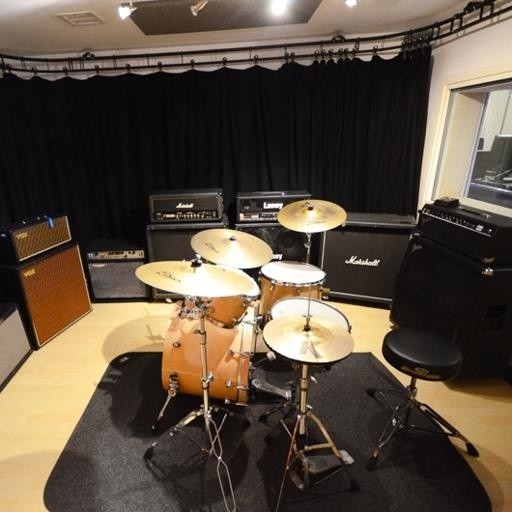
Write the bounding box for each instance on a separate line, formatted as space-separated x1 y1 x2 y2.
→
277 199 347 234
263 316 354 365
134 260 253 298
190 228 273 269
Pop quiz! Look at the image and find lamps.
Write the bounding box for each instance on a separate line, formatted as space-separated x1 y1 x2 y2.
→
117 5 139 20
189 0 209 17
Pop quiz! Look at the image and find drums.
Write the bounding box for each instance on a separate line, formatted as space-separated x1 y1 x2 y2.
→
162 300 259 400
260 260 327 315
270 297 351 334
200 265 260 328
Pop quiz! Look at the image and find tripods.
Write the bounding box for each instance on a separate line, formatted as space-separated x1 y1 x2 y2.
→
276 364 343 512
143 298 241 511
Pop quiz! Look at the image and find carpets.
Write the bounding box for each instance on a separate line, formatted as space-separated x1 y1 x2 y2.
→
40 353 492 511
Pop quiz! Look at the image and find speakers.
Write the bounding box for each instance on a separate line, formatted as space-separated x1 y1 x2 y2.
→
235 223 312 290
147 214 229 300
319 212 417 304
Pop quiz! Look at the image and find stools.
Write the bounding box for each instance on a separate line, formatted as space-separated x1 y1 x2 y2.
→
368 328 479 460
